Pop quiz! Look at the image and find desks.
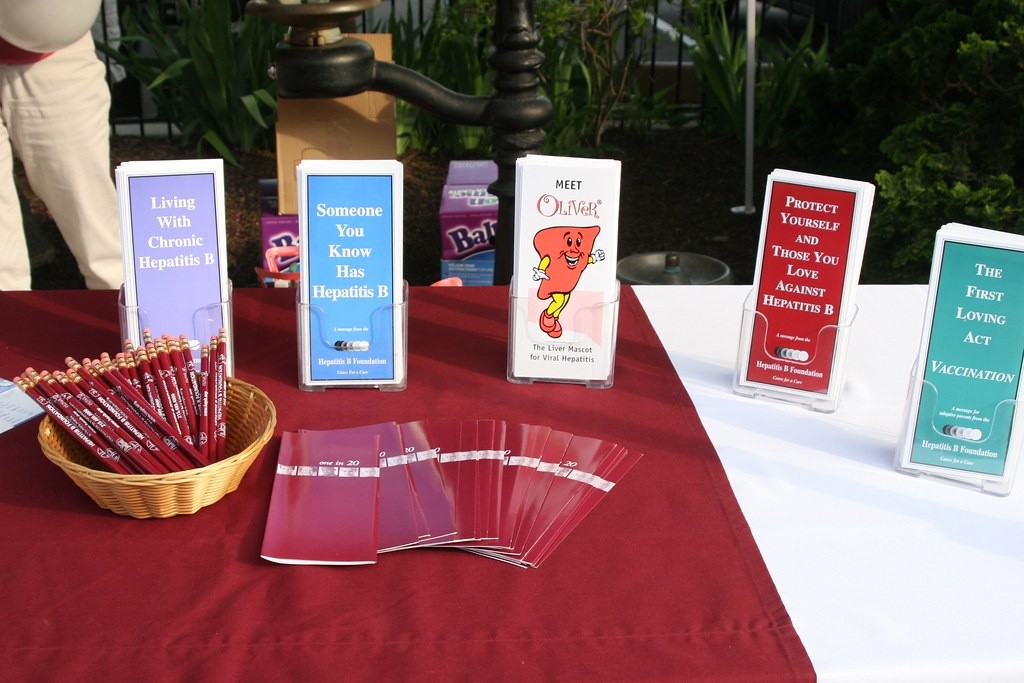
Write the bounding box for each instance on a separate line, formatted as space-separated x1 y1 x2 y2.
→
1 286 1024 683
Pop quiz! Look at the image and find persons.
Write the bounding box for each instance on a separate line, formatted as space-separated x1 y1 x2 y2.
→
0 0 131 291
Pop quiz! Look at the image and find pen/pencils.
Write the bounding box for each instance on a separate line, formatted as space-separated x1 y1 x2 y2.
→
12 326 228 474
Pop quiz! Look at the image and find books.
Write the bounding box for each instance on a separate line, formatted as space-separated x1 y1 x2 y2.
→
261 418 645 569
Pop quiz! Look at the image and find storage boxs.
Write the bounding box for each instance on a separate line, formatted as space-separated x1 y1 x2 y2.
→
733 285 859 416
441 154 500 285
506 272 620 392
295 281 408 393
276 31 398 216
259 212 300 287
118 278 235 378
893 354 1022 497
